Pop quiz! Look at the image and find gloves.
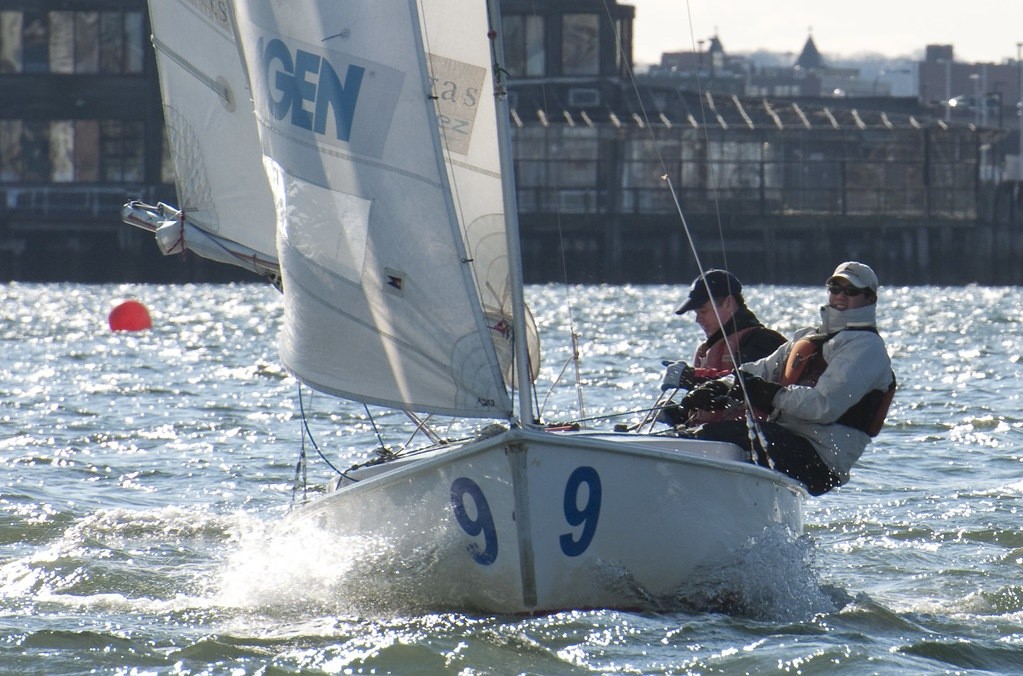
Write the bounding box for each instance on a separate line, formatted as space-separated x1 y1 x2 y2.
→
680 380 731 412
661 360 697 390
651 399 684 427
728 368 783 414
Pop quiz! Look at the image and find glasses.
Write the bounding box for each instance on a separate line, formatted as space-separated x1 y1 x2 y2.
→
828 282 873 296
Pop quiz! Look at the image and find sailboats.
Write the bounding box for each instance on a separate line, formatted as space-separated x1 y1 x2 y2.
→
119 1 814 616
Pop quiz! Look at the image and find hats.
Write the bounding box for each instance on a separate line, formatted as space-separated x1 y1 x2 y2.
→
826 261 879 295
675 268 741 315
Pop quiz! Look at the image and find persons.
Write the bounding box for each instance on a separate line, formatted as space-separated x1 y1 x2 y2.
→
721 261 896 496
660 268 788 451
